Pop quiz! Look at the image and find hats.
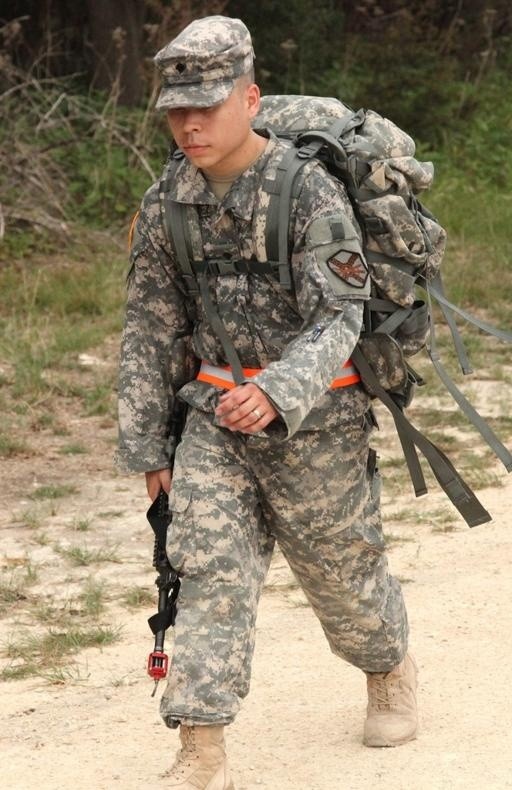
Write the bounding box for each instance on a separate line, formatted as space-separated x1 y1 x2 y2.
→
149 13 254 117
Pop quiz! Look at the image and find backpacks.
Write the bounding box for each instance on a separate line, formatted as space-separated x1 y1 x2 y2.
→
152 88 444 406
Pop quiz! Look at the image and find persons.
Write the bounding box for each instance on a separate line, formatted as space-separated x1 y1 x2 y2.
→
110 15 419 789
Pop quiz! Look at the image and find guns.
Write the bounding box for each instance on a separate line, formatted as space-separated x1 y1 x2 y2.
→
148 397 189 700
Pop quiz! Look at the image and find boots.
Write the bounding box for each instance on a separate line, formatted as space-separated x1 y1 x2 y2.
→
356 652 418 749
139 723 239 790
